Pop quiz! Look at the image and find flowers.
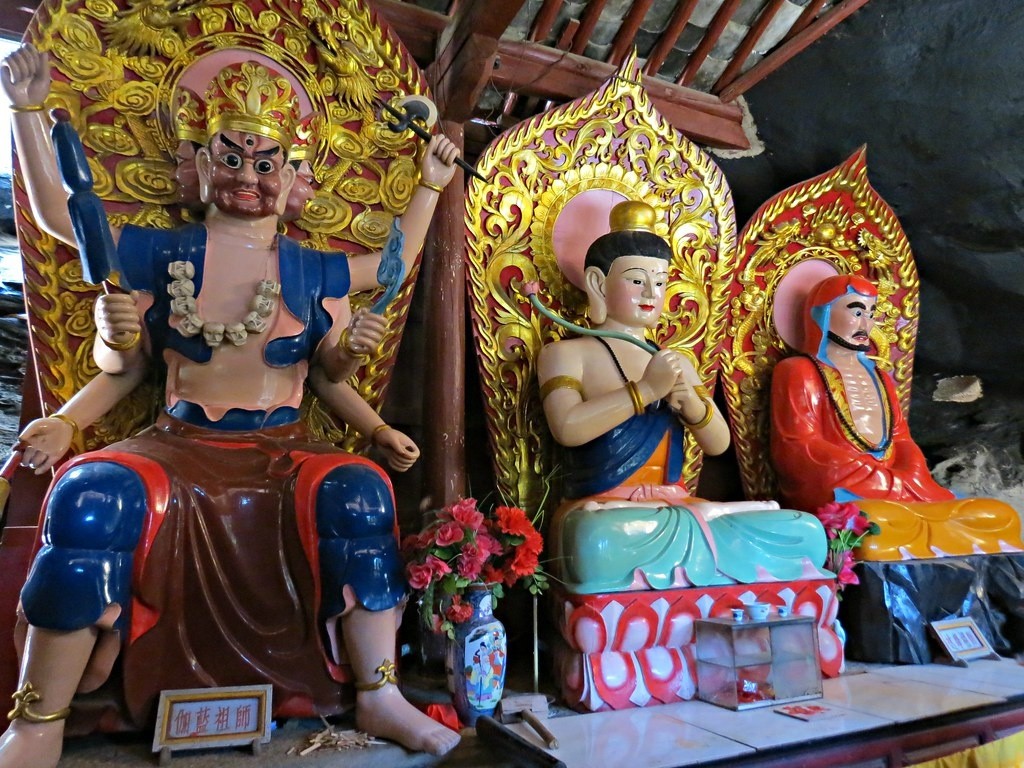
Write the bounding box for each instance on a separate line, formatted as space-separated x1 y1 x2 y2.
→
398 489 548 647
815 501 881 601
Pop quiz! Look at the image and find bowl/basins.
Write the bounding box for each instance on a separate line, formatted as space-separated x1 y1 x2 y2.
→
745 602 770 619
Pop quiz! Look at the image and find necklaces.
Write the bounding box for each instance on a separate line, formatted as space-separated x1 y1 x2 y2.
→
799 352 896 452
593 333 670 415
165 228 282 348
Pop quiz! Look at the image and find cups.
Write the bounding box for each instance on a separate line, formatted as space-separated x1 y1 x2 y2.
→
776 605 788 617
731 609 743 621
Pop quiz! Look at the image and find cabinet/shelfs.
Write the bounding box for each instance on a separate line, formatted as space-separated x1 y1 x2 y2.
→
694 610 825 712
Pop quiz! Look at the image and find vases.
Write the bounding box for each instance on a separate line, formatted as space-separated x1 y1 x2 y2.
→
445 581 508 727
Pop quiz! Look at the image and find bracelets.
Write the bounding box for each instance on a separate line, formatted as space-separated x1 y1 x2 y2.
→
628 381 645 416
418 178 445 194
341 328 368 359
96 331 141 351
679 394 715 431
49 414 79 443
373 424 392 448
9 104 45 113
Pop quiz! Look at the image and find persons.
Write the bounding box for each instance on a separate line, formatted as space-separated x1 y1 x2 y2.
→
2 37 468 768
770 273 1024 562
539 199 843 593
